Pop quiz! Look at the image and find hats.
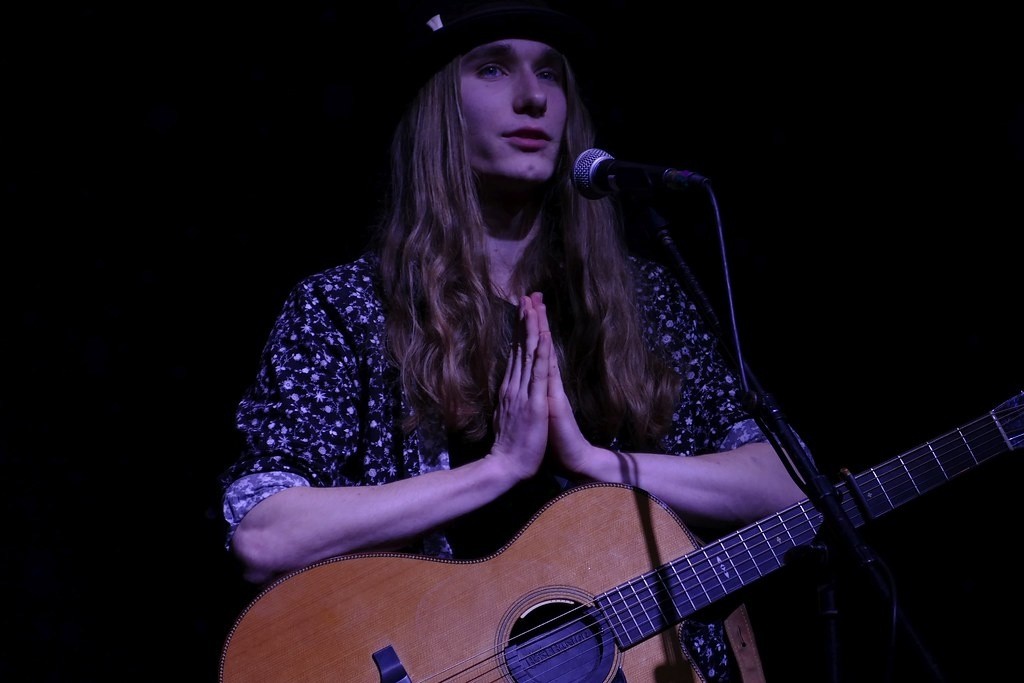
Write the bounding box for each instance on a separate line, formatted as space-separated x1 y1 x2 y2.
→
402 0 597 96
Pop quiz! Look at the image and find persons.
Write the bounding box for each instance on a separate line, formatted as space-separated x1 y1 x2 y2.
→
219 1 824 683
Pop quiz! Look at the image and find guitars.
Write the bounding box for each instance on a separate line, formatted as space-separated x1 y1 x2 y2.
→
212 386 1024 683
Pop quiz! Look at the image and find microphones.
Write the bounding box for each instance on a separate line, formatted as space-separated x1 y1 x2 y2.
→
570 149 709 201
